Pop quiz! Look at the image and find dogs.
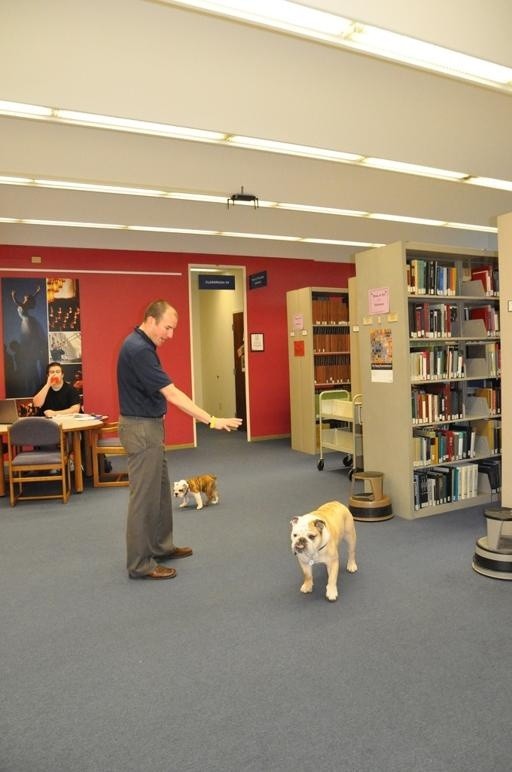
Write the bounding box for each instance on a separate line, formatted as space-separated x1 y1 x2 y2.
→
290 500 358 601
172 474 219 510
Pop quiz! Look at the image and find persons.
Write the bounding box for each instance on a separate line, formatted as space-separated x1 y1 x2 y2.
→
32 361 81 476
3 305 48 399
50 343 66 361
70 370 82 389
116 296 245 578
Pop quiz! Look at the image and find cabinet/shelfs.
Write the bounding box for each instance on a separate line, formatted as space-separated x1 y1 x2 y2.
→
286 241 502 521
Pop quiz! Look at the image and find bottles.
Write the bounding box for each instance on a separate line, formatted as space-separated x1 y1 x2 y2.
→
50 375 61 388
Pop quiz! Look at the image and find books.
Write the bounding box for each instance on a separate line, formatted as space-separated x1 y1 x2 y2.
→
52 413 96 421
406 260 501 511
314 300 351 446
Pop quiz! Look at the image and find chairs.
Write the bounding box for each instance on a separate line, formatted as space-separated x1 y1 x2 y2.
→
91 422 129 488
7 416 72 507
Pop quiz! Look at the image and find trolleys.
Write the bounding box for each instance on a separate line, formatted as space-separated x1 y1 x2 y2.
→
317 389 363 481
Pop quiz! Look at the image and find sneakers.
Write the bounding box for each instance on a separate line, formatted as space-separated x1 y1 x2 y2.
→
49 469 61 476
68 458 85 474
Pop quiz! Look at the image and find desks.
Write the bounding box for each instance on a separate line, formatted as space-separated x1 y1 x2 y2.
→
0 416 109 497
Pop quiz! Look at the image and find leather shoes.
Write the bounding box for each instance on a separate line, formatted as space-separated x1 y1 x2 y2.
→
153 546 193 561
142 564 177 580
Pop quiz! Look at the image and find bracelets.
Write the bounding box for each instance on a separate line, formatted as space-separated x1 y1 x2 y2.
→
211 415 216 431
208 416 214 427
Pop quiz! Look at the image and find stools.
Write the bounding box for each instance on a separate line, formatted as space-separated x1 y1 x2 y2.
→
473 508 512 581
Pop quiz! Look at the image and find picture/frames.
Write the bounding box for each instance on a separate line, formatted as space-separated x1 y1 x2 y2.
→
250 333 264 352
